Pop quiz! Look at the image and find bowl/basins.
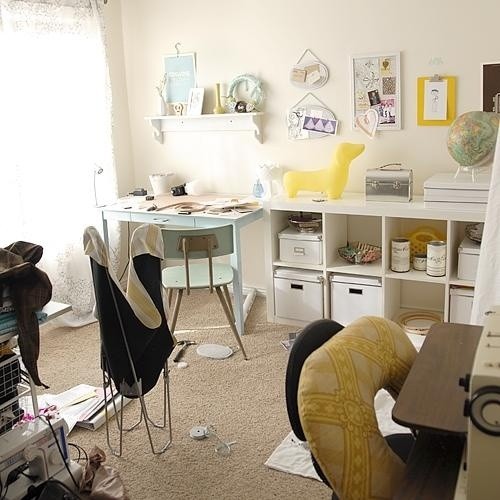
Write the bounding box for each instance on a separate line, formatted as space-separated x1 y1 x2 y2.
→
287 212 322 234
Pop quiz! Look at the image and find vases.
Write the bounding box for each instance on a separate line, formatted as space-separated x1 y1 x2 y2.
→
213 82 225 113
156 97 167 116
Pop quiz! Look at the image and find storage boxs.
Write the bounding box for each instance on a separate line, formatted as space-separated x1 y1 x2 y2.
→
274 269 323 322
277 226 324 265
450 287 475 325
457 234 485 280
331 273 383 328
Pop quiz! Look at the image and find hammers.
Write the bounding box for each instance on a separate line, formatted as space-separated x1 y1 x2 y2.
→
173 340 196 362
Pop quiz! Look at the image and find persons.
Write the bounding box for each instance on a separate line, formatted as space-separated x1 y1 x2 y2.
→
370 91 395 124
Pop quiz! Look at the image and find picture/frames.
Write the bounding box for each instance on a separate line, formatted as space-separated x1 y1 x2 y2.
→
351 52 401 139
186 87 205 116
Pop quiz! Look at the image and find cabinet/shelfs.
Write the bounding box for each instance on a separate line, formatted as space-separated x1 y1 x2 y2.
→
263 199 491 328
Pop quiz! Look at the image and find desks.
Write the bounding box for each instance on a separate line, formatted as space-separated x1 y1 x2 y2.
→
0 300 74 442
388 322 482 500
100 191 263 334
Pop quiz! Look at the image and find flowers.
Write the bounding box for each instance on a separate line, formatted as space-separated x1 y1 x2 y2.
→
153 73 168 96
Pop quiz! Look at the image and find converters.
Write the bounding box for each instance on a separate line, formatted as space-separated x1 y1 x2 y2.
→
133 188 147 196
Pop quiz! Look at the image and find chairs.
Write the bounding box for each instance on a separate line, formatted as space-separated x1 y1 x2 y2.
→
160 221 248 360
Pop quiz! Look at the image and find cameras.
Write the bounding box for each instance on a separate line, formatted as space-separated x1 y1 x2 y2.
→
170 185 187 197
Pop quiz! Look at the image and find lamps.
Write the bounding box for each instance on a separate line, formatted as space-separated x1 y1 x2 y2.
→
93 165 118 208
